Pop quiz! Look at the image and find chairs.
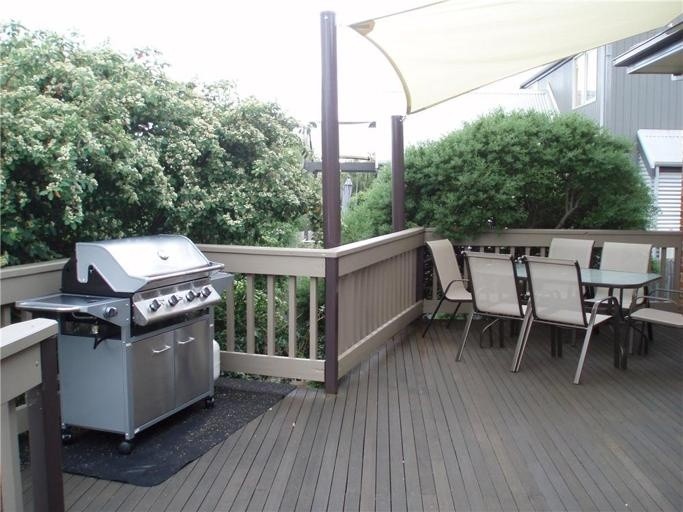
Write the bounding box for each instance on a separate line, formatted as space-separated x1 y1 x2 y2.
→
422 238 683 385
